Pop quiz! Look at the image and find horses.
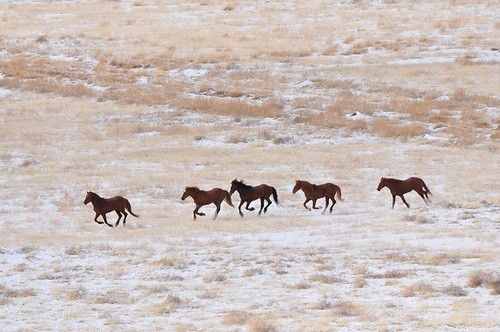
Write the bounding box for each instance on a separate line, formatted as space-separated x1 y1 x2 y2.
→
228 179 280 219
291 179 345 214
83 190 140 228
180 186 235 221
377 176 433 208
